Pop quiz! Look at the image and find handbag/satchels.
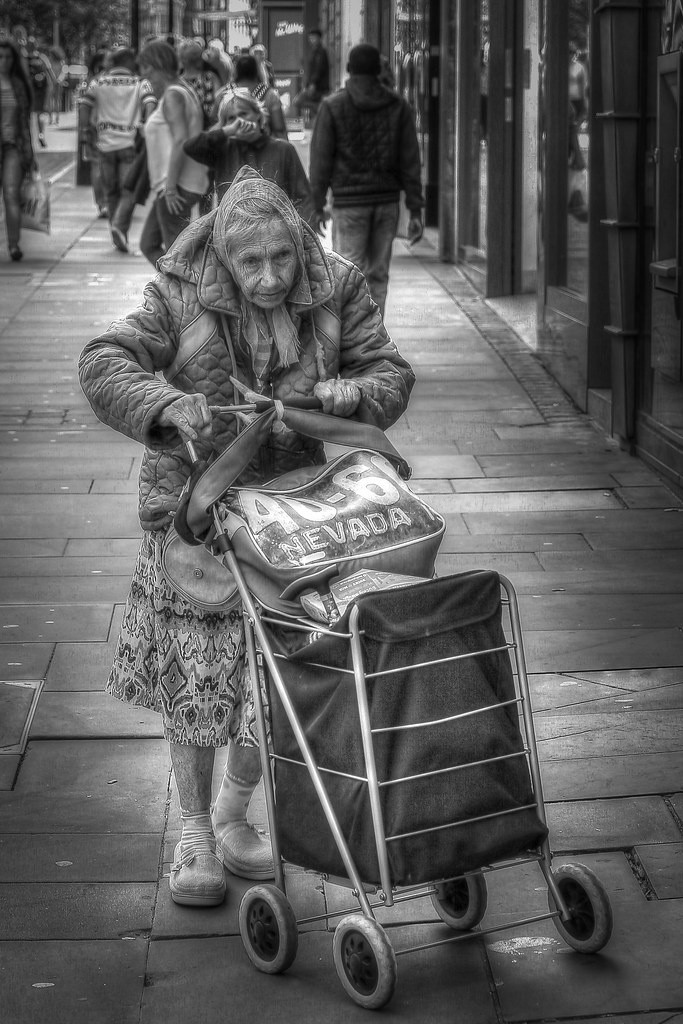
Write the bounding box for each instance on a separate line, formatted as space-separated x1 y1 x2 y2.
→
159 523 244 611
185 405 445 616
19 166 50 233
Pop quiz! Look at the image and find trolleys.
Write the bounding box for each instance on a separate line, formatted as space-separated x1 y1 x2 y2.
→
177 397 613 1011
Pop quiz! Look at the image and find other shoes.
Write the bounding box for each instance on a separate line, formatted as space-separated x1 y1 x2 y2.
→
112 226 129 252
99 206 110 217
210 805 277 880
169 842 226 907
9 244 23 261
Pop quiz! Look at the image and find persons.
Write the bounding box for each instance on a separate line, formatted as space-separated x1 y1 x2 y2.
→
184 87 313 223
134 41 210 273
78 178 417 906
293 28 331 118
0 40 40 263
23 37 69 147
72 36 288 252
306 43 424 322
569 44 588 172
